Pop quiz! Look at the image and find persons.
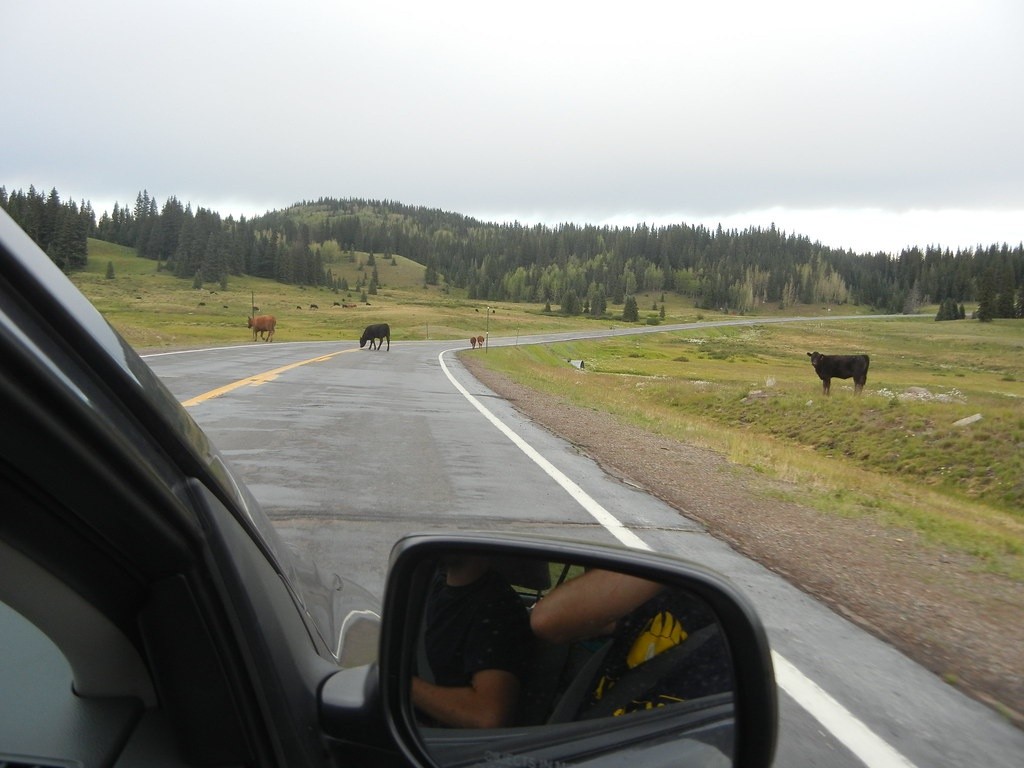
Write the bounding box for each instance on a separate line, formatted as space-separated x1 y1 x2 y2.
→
407 546 527 731
477 334 485 348
526 564 732 726
470 336 477 349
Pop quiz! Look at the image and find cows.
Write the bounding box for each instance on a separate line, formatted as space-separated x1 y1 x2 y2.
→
254 307 259 310
247 315 276 342
223 305 228 308
470 337 477 350
475 308 479 312
359 322 390 352
806 351 870 398
296 302 372 310
477 335 485 348
198 302 206 306
492 309 496 313
